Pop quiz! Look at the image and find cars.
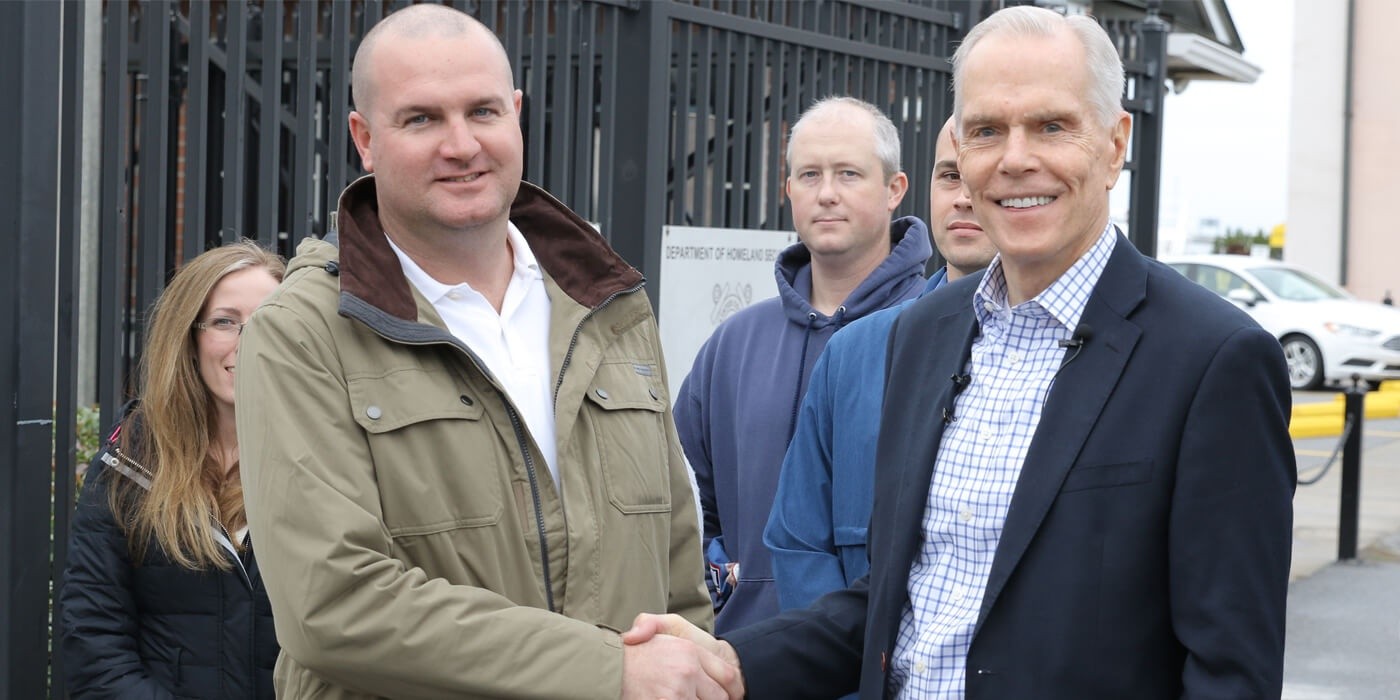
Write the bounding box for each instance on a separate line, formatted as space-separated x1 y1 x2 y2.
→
1155 252 1400 392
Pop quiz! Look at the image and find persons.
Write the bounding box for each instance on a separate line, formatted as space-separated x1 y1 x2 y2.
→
672 95 934 643
233 4 744 700
615 5 1298 700
763 113 999 613
55 240 291 700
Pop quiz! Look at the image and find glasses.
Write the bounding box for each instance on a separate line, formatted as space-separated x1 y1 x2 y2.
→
194 315 246 339
